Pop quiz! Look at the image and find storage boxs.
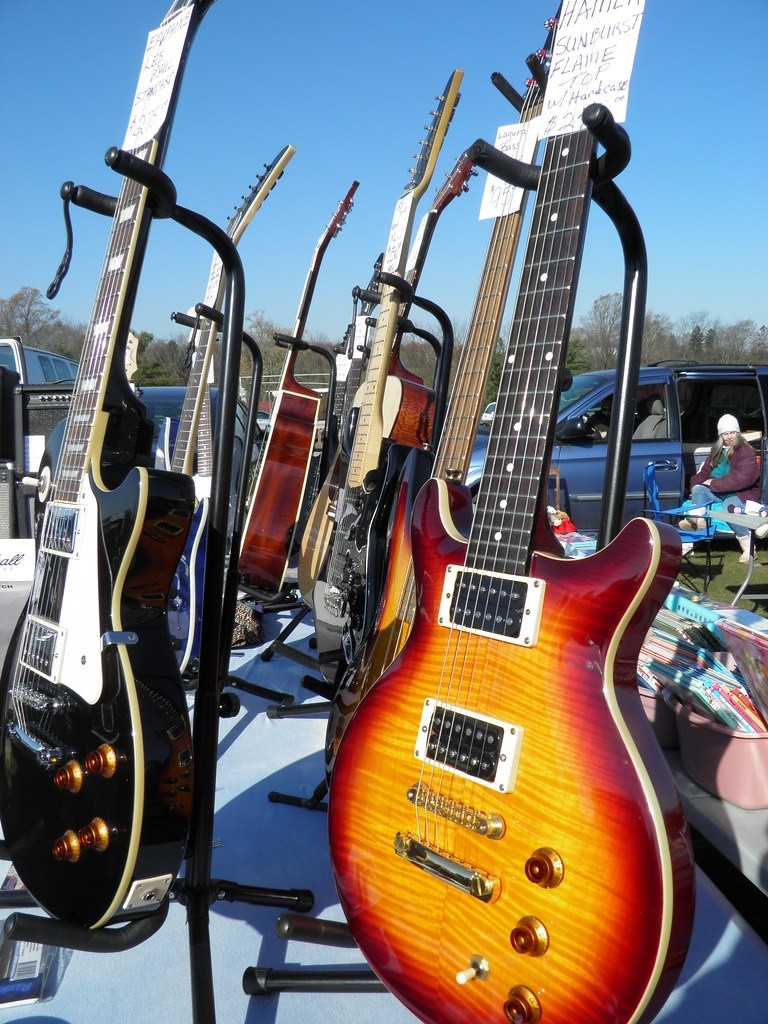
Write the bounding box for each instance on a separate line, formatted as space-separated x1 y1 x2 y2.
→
638 686 677 750
661 686 768 809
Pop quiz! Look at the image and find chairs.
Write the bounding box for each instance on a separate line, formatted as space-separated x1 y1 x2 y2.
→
642 460 717 594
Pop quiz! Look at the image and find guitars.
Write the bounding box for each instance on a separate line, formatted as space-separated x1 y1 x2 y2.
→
297 68 479 687
324 2 568 784
151 144 298 691
326 129 698 1023
1 0 215 934
237 179 361 594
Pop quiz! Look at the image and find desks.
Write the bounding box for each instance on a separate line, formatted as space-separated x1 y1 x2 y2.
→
708 511 768 608
542 532 768 946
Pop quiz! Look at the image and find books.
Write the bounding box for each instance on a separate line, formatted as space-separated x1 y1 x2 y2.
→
557 532 768 733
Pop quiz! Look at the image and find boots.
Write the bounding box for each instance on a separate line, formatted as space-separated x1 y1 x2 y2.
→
679 507 706 530
736 531 756 562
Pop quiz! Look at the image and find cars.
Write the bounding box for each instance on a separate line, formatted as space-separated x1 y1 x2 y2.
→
462 357 768 536
32 385 267 514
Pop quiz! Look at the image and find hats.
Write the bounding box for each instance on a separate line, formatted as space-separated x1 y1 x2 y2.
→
718 414 740 435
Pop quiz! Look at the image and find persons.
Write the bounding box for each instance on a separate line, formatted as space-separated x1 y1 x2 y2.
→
679 415 759 563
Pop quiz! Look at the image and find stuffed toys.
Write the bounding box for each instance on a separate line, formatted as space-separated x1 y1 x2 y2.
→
548 506 576 535
